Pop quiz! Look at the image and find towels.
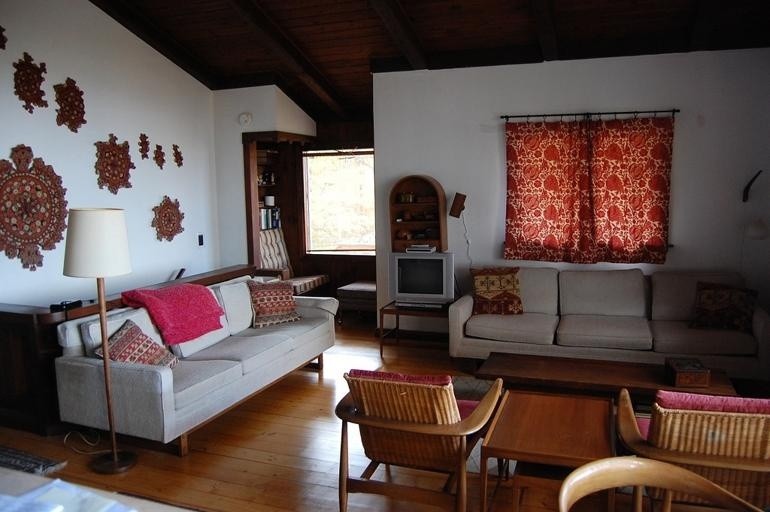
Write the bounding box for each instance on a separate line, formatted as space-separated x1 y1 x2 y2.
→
120 281 226 349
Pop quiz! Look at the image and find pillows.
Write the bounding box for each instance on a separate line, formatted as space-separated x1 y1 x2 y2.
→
92 317 180 373
686 278 761 337
246 277 306 330
464 264 525 318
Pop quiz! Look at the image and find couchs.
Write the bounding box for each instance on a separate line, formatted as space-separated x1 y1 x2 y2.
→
443 263 770 383
52 272 344 461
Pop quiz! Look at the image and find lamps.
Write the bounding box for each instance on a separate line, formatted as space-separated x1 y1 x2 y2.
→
737 215 770 278
60 204 148 476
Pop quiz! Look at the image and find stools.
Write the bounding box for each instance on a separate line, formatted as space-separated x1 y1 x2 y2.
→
332 280 377 326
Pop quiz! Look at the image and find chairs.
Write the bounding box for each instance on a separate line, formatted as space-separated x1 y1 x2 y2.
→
257 227 330 297
613 382 768 511
332 360 507 511
553 454 766 512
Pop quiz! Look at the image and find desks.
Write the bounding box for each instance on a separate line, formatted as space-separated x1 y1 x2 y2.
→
477 386 619 511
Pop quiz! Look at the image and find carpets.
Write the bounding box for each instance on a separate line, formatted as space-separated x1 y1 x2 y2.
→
448 373 517 481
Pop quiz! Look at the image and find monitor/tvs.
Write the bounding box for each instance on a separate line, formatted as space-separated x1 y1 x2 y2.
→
388 252 454 309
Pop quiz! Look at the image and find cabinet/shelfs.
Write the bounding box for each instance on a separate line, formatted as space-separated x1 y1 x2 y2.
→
388 174 449 254
376 298 459 358
253 146 283 210
